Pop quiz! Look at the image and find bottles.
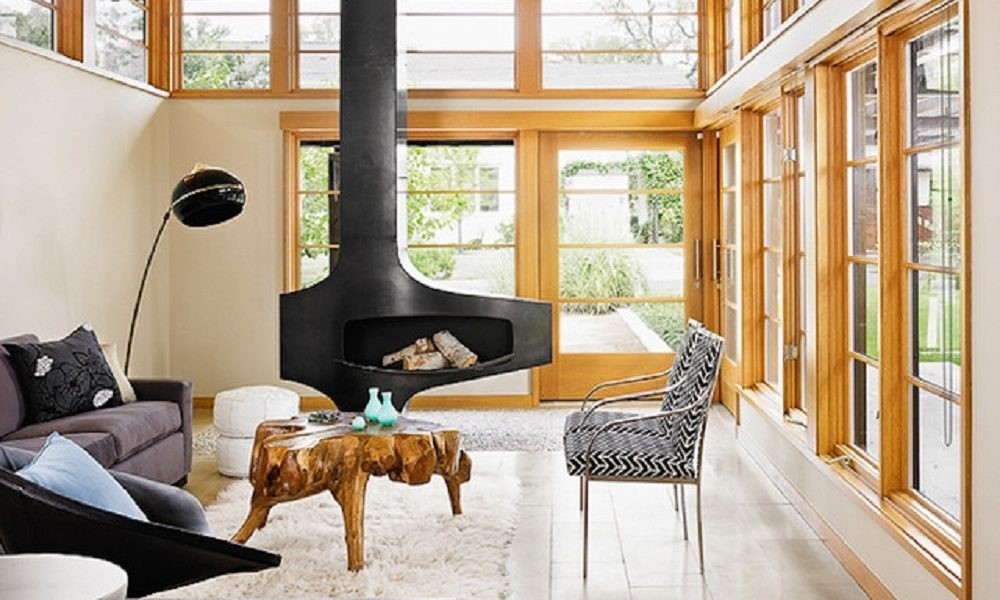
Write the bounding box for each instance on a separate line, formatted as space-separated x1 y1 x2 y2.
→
364 387 382 424
378 392 398 427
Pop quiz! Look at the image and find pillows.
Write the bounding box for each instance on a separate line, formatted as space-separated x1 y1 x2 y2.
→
6 322 152 521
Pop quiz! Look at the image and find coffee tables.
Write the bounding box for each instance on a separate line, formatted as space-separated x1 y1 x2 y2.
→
232 412 473 569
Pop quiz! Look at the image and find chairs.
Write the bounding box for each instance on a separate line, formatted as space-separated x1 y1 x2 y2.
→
0 446 210 535
563 318 724 578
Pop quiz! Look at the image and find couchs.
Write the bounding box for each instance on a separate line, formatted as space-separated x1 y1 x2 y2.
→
0 333 192 488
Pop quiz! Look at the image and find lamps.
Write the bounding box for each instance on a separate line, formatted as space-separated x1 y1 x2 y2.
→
124 166 246 374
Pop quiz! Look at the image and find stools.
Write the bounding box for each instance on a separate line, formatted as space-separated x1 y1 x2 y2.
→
215 386 300 477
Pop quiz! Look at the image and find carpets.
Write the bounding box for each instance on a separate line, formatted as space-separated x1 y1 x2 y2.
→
138 451 516 600
